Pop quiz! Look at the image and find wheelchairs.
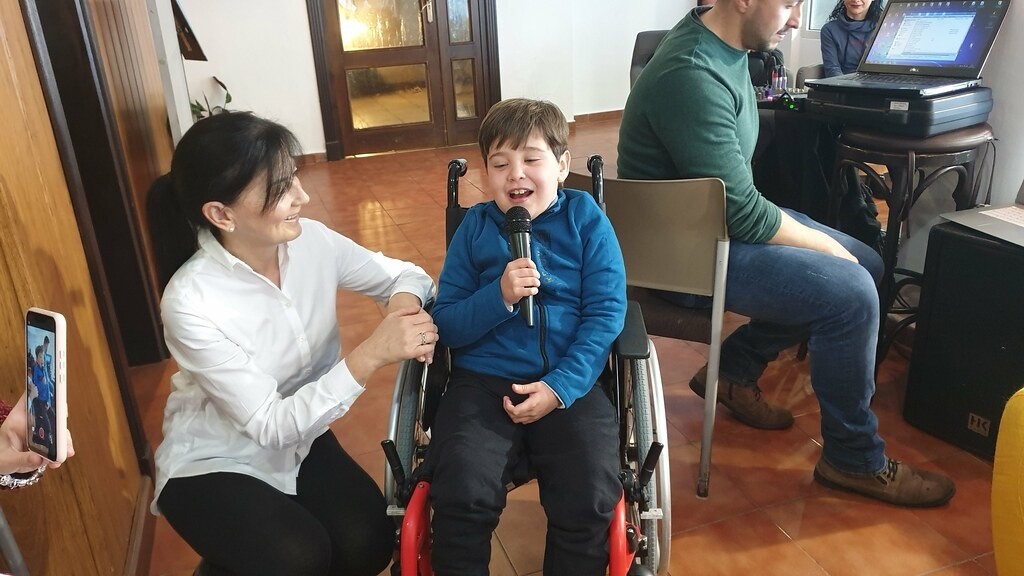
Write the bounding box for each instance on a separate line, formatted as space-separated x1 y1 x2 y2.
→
373 152 676 575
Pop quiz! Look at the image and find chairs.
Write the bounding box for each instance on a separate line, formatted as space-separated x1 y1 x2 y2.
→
563 170 729 497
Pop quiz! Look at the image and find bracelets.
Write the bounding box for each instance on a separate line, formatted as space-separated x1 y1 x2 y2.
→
0 464 46 490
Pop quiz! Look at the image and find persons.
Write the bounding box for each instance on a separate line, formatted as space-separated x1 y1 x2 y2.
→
27 335 57 460
0 388 76 487
427 98 627 576
145 112 439 576
616 0 957 509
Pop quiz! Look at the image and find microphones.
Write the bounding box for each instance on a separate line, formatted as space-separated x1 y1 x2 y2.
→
503 205 536 328
42 364 44 378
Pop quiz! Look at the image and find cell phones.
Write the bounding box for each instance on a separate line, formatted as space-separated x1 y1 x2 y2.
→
24 307 68 464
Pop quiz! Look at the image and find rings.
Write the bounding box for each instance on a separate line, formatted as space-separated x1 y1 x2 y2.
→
421 331 426 345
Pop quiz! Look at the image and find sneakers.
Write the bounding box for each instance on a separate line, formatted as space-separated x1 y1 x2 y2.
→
689 361 795 431
813 458 956 508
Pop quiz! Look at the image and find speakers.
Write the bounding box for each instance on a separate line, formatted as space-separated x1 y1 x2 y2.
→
902 221 1024 460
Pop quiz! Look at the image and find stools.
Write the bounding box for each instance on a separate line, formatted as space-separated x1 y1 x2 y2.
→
796 123 998 405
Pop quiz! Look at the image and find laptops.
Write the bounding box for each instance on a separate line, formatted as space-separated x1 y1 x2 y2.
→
940 180 1024 248
803 0 1011 99
